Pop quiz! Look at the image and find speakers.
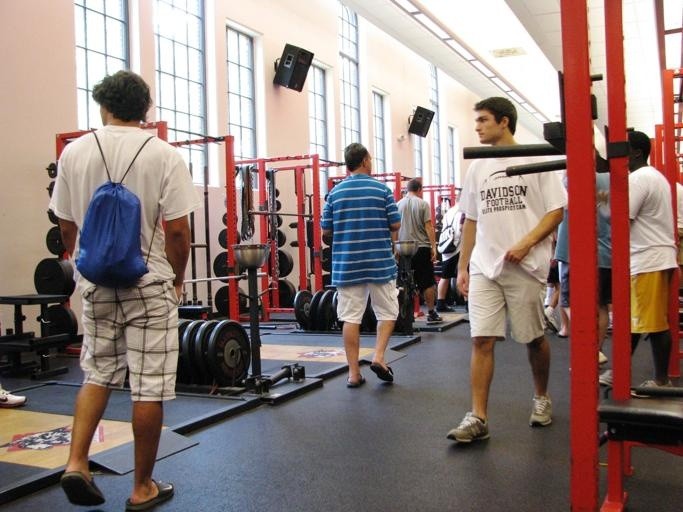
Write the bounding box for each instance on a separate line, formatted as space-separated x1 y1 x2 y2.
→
408 105 435 138
273 44 314 92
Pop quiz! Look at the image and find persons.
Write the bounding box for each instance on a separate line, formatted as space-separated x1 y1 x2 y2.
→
0 377 28 409
47 69 204 511
397 178 444 324
436 96 682 443
321 144 401 387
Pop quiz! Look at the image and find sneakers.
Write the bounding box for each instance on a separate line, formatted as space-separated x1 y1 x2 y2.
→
528 394 553 427
427 309 442 321
447 412 490 442
544 306 560 332
631 380 673 398
0 388 27 408
599 369 612 386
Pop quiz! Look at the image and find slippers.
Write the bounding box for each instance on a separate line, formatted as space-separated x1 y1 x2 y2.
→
370 362 393 381
61 471 105 505
347 374 365 388
436 299 455 312
126 479 174 511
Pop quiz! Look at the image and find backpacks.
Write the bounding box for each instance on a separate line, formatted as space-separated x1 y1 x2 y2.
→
75 128 160 286
437 210 460 254
